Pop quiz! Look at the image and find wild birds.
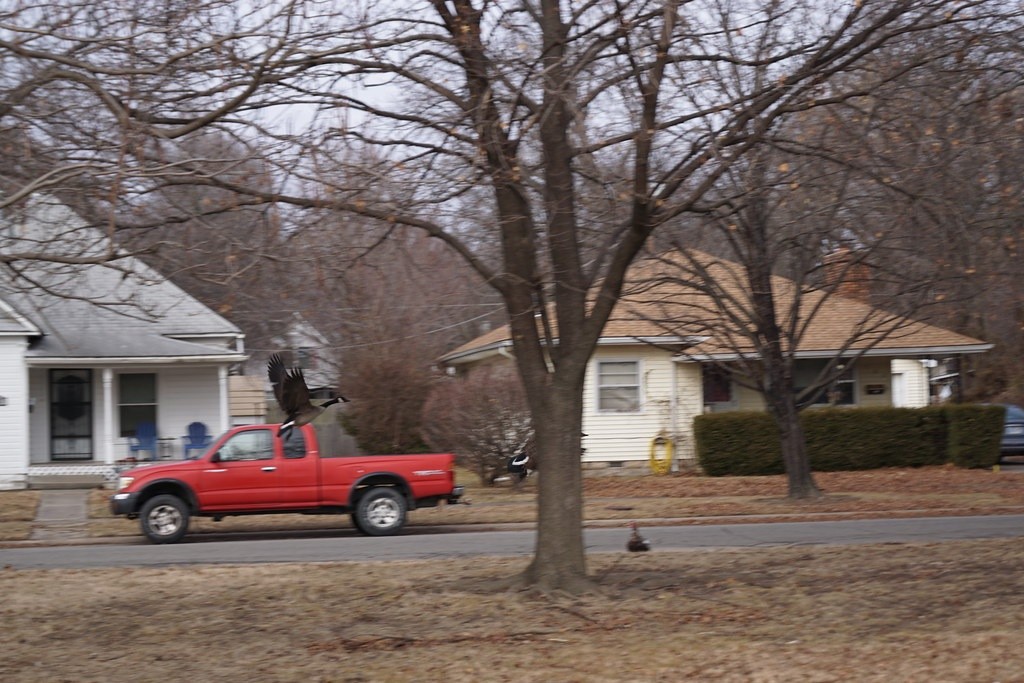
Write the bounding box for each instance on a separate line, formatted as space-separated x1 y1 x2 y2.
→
627 523 649 552
268 353 351 441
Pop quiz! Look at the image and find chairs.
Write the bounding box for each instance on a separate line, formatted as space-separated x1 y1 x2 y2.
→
181 422 213 460
126 422 157 460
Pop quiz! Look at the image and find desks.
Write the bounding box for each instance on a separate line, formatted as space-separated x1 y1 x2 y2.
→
155 438 176 457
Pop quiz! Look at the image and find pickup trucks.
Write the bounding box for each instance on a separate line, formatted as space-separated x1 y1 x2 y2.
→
109 419 465 536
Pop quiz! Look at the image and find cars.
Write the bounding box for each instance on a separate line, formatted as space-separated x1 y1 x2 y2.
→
993 404 1024 462
